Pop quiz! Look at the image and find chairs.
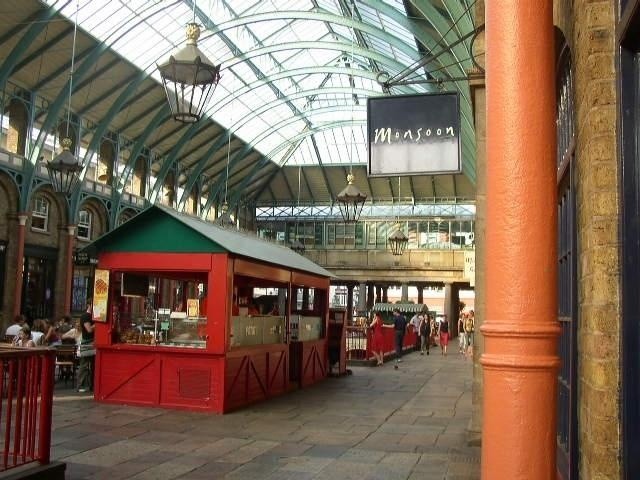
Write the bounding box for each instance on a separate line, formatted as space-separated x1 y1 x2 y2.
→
0 334 78 389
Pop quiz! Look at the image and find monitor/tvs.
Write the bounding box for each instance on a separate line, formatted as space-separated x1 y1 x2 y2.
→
122 272 149 297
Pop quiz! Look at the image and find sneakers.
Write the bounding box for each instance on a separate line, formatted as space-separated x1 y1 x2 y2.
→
377 361 384 368
395 358 402 362
459 350 466 354
420 351 429 355
441 351 447 355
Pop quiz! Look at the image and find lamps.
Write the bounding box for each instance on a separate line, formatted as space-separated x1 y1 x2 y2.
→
154 0 224 124
45 0 87 197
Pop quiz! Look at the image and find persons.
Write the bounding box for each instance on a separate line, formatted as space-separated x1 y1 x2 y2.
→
74 296 97 393
266 301 280 316
364 306 474 366
3 312 84 393
